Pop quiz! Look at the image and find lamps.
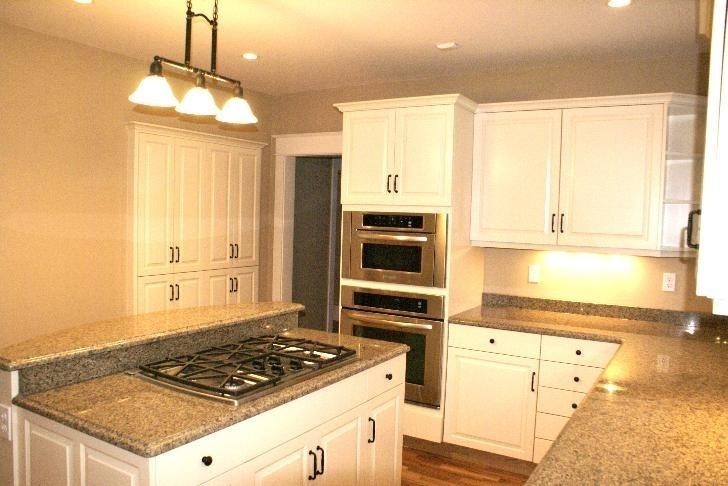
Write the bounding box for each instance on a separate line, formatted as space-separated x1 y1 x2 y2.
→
128 0 261 125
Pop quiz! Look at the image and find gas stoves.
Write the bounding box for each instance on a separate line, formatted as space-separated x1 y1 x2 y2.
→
137 335 359 406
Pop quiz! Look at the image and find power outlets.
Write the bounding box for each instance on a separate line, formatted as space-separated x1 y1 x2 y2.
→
0 403 14 443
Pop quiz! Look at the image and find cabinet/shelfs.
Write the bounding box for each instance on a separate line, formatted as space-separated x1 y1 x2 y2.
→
14 353 407 486
694 2 727 316
334 94 485 445
469 92 706 259
125 121 268 315
442 322 620 466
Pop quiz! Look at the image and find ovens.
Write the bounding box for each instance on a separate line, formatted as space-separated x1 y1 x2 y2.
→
343 211 449 289
340 285 444 410
